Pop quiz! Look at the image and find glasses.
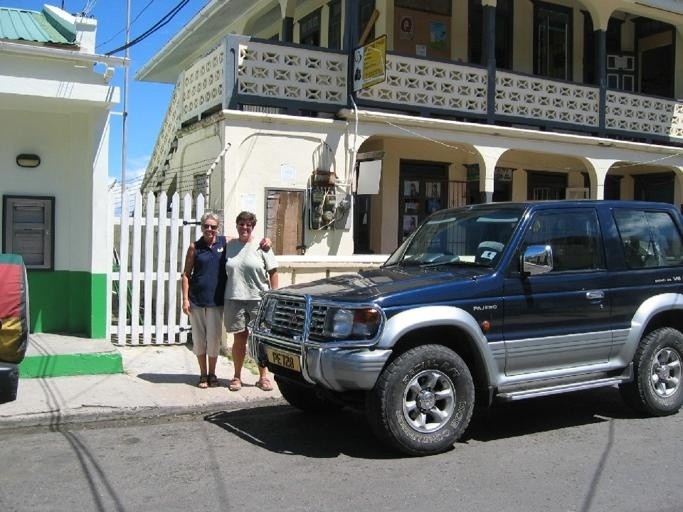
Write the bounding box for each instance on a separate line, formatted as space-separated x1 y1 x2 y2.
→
202 224 218 230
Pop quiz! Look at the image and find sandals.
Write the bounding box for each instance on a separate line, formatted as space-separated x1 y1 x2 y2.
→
198 375 220 388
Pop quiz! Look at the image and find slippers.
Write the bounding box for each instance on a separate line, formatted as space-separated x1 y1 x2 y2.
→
228 378 241 390
256 377 273 390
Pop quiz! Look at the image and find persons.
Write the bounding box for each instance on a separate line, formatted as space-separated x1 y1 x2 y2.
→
181 211 272 389
222 211 278 392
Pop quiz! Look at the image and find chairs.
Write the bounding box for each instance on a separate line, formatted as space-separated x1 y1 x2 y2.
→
557 244 584 270
475 240 503 266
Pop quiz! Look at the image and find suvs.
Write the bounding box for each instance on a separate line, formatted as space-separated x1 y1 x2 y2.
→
247 200 683 453
